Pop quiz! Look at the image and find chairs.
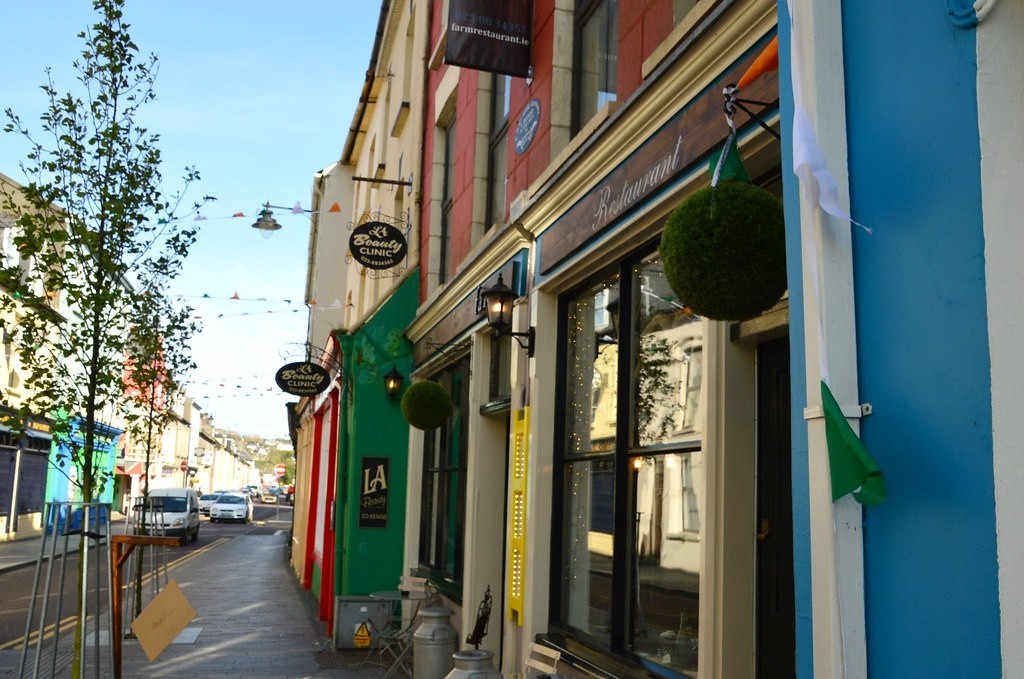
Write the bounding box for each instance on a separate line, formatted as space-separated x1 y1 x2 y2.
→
524 643 561 674
378 594 440 679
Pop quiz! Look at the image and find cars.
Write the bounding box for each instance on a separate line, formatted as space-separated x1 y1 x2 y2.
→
214 481 295 506
197 491 223 517
210 492 254 525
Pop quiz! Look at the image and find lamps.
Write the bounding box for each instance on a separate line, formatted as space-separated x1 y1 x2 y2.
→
383 364 404 402
481 273 534 357
252 202 321 230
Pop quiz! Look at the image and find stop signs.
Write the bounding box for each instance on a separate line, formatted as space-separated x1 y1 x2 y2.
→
274 463 286 476
180 461 188 472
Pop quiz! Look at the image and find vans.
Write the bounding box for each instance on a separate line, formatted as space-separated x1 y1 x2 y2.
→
132 488 200 547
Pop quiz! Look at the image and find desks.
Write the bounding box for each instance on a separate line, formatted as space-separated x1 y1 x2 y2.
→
362 590 438 671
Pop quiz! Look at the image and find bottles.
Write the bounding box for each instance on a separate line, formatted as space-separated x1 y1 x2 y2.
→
674 612 698 671
443 648 504 679
413 606 459 679
